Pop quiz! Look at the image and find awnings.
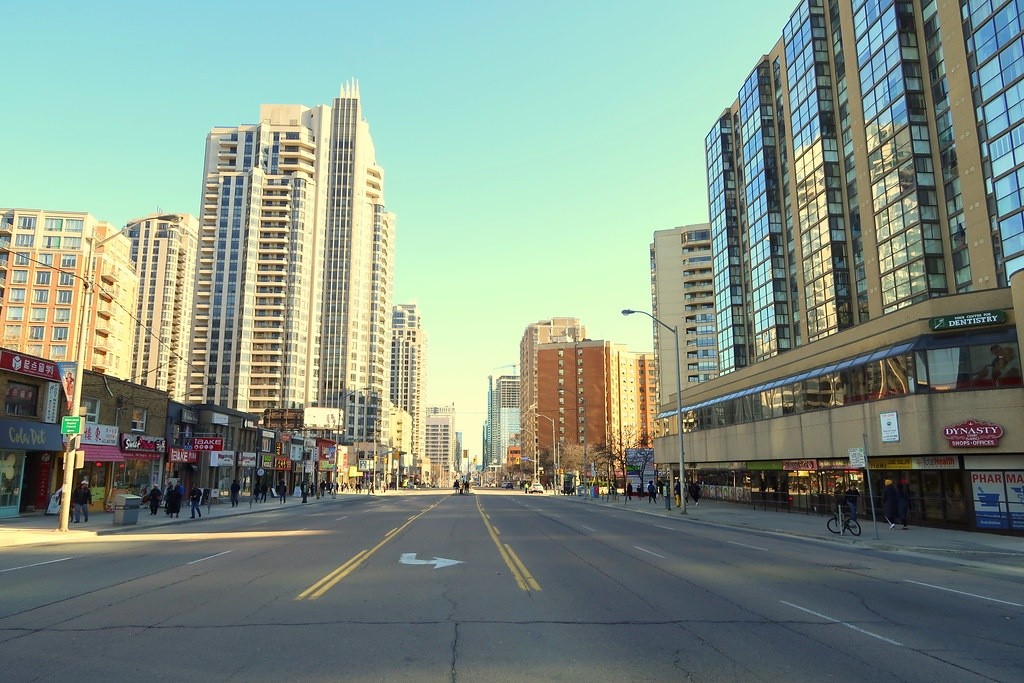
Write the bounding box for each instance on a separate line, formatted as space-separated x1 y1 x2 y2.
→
64 443 126 462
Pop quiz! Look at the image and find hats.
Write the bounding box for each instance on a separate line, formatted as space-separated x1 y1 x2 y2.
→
154 483 158 487
81 480 89 485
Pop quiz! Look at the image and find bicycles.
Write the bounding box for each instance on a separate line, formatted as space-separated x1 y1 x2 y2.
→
826 505 863 536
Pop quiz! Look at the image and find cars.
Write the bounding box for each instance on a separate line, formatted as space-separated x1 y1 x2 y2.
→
528 483 544 494
501 481 513 489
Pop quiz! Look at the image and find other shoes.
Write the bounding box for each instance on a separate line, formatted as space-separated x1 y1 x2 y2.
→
889 523 895 529
74 521 79 523
84 519 88 522
902 526 908 529
170 513 201 518
696 502 699 506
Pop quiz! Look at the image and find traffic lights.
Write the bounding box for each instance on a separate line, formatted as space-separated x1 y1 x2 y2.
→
473 457 477 464
516 458 519 465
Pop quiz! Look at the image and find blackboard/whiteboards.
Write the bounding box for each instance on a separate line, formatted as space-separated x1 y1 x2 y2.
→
202 488 212 501
294 487 301 496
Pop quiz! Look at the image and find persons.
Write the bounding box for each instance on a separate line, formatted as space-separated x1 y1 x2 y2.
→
254 482 267 503
846 484 860 520
149 484 161 515
674 481 701 507
973 345 1020 380
627 482 632 500
647 481 657 503
231 480 240 507
307 480 372 497
189 483 202 518
453 476 469 493
637 485 640 493
57 480 92 523
279 480 286 504
65 372 74 390
880 485 908 530
164 480 184 518
524 483 528 493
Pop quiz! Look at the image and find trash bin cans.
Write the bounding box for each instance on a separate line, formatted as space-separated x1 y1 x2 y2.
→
113 494 142 526
578 486 584 497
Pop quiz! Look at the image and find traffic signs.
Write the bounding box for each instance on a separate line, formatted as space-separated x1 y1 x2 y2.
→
61 416 80 434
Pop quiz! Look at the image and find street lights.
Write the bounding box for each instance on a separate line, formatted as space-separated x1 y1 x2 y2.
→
519 427 536 483
534 413 557 497
330 387 372 500
59 213 184 535
558 385 587 501
620 308 687 516
